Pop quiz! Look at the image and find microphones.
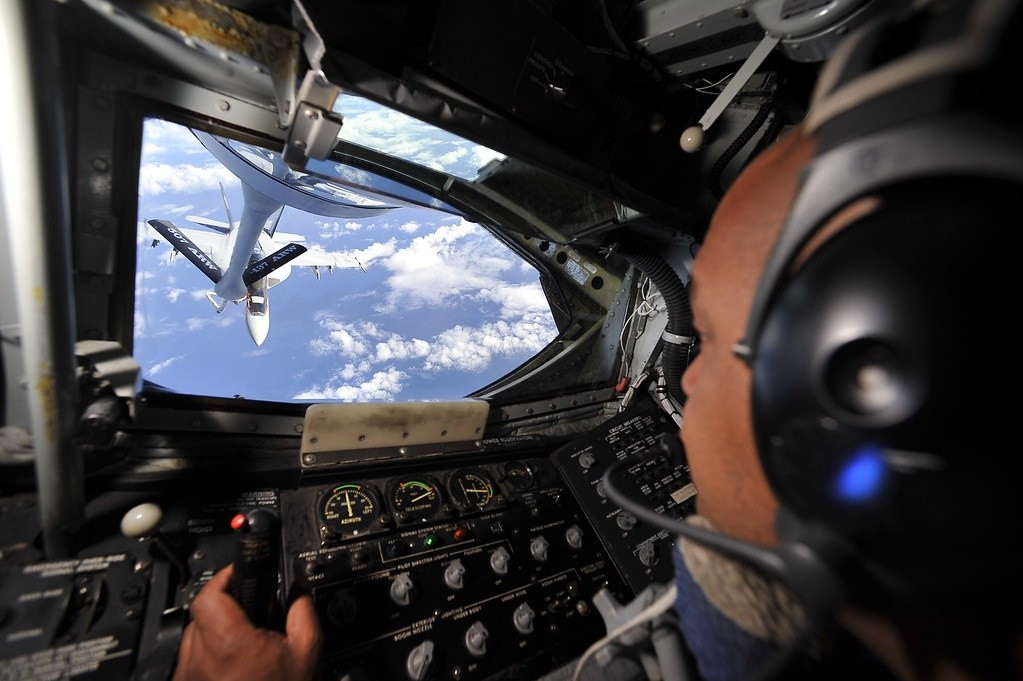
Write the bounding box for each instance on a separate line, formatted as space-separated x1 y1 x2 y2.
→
602 443 788 583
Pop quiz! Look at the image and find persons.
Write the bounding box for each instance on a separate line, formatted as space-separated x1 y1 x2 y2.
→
172 107 1023 681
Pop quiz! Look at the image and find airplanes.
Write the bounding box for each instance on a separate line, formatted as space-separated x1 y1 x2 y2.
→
135 179 369 350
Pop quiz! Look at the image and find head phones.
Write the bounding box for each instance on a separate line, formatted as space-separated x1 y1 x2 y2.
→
733 0 1022 619
